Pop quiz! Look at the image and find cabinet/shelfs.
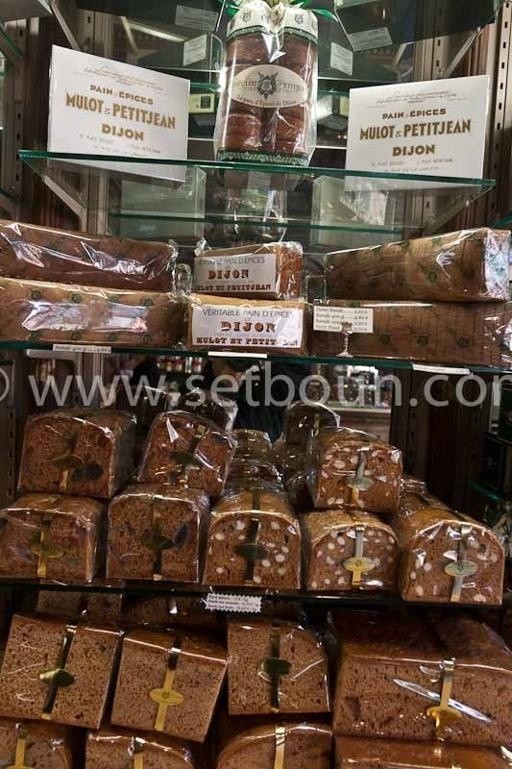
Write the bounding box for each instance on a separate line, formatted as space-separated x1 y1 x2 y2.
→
0 144 511 612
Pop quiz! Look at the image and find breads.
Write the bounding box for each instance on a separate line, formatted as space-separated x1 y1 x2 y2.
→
0 277 184 350
0 218 182 292
0 589 512 769
309 296 511 375
216 30 320 159
0 403 507 611
322 227 512 306
191 241 305 303
